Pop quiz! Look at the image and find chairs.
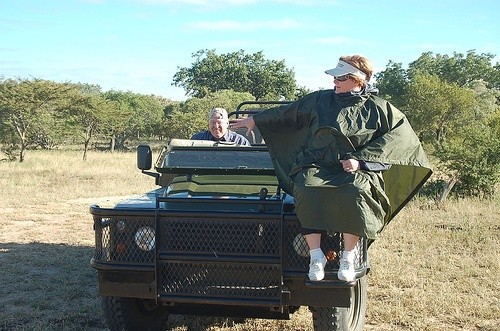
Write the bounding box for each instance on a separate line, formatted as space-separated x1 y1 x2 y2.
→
228 127 255 144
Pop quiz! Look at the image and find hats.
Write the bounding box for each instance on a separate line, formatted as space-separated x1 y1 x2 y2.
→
325 60 367 81
210 108 228 119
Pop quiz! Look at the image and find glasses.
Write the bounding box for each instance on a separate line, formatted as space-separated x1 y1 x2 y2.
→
334 75 350 81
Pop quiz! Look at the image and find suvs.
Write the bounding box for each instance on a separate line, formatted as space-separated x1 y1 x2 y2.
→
88 100 368 331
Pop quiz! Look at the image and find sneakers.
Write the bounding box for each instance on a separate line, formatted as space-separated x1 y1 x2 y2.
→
338 258 356 282
308 255 327 280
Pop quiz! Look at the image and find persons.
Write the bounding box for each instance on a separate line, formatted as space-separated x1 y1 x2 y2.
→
190 108 250 146
230 55 433 281
